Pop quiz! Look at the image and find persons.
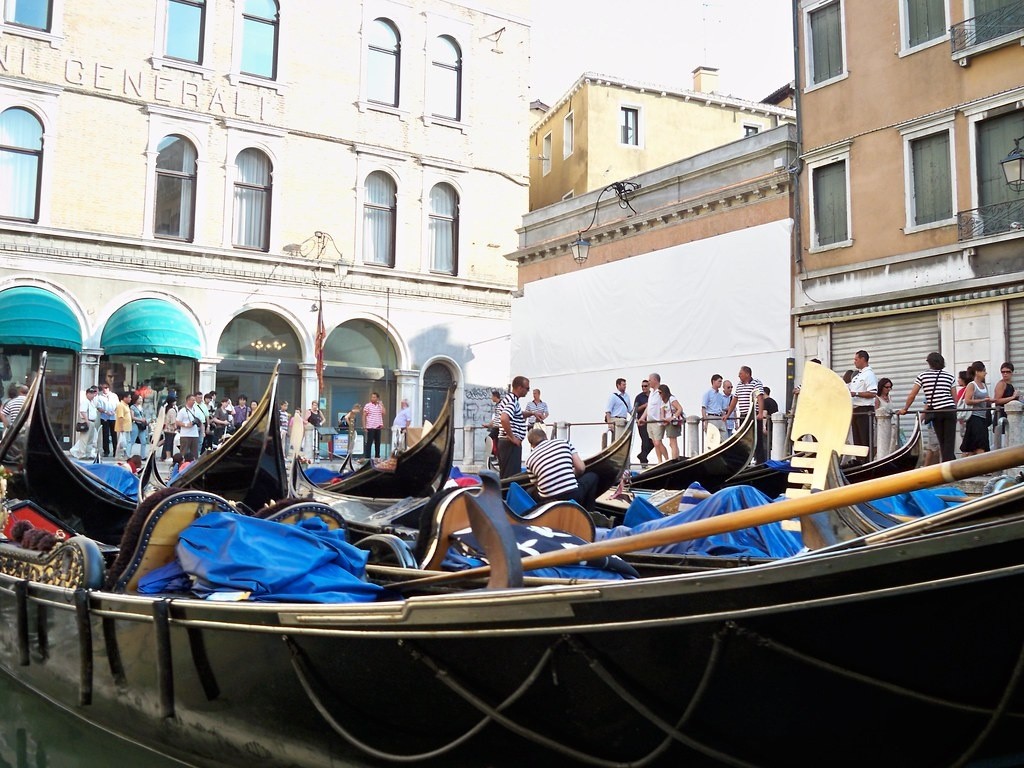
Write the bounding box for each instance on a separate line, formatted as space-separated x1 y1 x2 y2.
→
103 362 126 394
114 380 258 483
279 400 289 462
605 378 634 432
633 380 655 469
303 400 327 459
701 365 778 466
482 375 600 511
393 398 412 451
638 373 684 463
920 396 942 468
957 361 1021 458
362 392 386 458
345 403 362 447
792 349 893 465
0 381 29 440
69 382 120 460
289 407 309 427
896 352 959 463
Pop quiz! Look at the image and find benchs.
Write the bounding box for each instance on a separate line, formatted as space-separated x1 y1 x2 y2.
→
419 482 598 573
108 491 351 602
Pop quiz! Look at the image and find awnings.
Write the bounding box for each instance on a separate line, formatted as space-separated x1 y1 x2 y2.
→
0 286 83 354
100 298 202 360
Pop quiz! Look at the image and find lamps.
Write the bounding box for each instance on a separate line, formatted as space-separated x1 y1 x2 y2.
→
316 230 349 280
570 181 642 264
1001 135 1024 194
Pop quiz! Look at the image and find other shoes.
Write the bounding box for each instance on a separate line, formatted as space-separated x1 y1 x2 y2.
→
637 453 649 463
113 452 116 457
104 454 108 457
164 457 173 462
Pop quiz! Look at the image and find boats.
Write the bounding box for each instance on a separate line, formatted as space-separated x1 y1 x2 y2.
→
0 350 1024 768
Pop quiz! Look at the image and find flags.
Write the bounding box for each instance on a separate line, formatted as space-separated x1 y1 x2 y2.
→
315 297 325 395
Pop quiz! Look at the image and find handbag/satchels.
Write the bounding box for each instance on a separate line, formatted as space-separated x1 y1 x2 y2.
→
925 406 935 425
956 382 976 421
671 411 686 425
137 422 147 430
76 422 89 432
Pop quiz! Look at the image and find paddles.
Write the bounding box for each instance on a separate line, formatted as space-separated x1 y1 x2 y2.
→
382 443 1024 594
365 563 612 586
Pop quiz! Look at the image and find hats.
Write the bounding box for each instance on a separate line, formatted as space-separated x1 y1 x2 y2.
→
169 392 178 398
165 395 177 403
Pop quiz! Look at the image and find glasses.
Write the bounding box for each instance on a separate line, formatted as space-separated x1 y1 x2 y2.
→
658 391 662 394
521 385 530 391
1001 370 1013 374
641 385 649 388
883 385 892 389
727 386 733 389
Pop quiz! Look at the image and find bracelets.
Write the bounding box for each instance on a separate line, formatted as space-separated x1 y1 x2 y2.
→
902 406 907 410
672 415 676 419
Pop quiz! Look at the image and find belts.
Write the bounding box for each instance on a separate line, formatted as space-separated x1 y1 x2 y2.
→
708 413 722 416
